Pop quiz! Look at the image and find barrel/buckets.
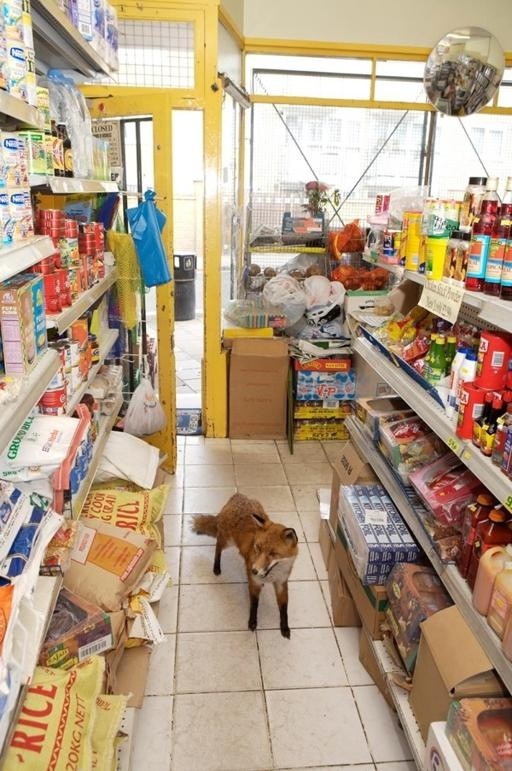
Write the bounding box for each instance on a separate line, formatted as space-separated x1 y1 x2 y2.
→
472 544 511 661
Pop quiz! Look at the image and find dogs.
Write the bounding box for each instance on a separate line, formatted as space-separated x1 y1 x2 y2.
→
188 492 298 640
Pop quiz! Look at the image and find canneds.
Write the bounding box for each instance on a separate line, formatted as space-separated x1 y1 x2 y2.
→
28 209 106 415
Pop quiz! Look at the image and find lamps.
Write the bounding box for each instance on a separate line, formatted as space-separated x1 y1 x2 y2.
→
220 72 251 111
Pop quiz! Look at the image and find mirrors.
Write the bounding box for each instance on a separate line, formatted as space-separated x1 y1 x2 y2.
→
423 27 506 116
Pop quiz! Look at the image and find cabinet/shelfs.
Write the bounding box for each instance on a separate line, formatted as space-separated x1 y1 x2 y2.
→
315 245 512 771
0 0 122 771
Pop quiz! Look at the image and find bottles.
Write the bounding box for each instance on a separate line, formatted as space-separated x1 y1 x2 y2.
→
421 327 512 481
52 115 74 179
454 489 512 596
442 174 512 300
91 334 100 365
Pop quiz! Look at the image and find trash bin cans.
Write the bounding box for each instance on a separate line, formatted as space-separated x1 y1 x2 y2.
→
173 255 197 321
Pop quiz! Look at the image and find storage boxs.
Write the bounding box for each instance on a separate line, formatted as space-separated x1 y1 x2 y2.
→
228 339 288 441
292 330 510 771
